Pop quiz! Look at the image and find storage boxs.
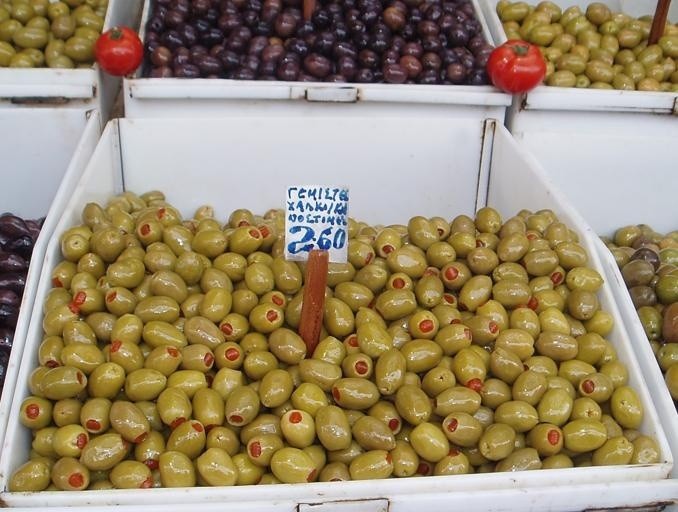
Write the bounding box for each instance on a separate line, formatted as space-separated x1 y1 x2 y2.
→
0 106 105 450
118 0 513 118
1 1 142 133
591 223 678 410
0 114 677 511
483 2 678 241
